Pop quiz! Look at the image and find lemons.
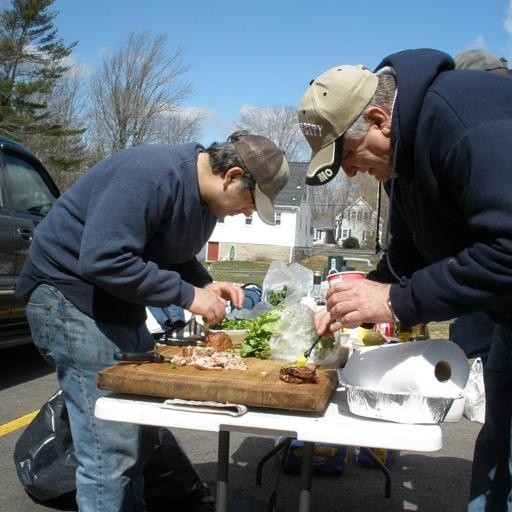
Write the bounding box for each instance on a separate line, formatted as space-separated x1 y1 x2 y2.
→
362 330 387 346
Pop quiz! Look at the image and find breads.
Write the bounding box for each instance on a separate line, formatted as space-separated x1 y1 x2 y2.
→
183 333 233 357
279 366 317 384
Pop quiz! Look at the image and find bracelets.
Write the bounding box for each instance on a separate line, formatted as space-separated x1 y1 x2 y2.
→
387 301 399 323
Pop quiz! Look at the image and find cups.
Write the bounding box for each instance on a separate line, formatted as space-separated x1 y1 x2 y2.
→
326 271 366 285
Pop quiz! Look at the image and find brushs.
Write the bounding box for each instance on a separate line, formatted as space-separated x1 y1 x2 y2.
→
296 319 335 367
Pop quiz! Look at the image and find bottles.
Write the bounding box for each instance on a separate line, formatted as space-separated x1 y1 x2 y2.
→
311 269 324 302
329 259 338 274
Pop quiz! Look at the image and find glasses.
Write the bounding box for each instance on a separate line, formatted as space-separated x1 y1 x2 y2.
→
246 189 257 215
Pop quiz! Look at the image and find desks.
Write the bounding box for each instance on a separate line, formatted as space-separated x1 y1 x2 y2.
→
93 285 444 512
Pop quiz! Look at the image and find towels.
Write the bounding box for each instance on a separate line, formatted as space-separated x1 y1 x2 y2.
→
158 395 249 417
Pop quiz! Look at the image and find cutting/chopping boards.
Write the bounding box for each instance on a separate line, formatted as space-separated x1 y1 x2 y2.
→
95 336 349 411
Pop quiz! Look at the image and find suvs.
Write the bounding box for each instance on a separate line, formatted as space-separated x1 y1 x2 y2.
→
0 135 60 371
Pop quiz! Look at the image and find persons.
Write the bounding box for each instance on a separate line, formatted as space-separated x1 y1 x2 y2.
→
297 47 511 511
14 130 290 512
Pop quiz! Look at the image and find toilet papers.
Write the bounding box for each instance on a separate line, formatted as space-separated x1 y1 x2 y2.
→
343 338 471 400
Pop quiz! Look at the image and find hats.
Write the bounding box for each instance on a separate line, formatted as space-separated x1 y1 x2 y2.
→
298 64 379 186
234 134 289 225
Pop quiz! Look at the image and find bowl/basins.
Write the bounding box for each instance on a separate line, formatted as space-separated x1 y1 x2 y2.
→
207 325 250 346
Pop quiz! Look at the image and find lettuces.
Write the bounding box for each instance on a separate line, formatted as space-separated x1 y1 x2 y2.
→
204 309 281 359
